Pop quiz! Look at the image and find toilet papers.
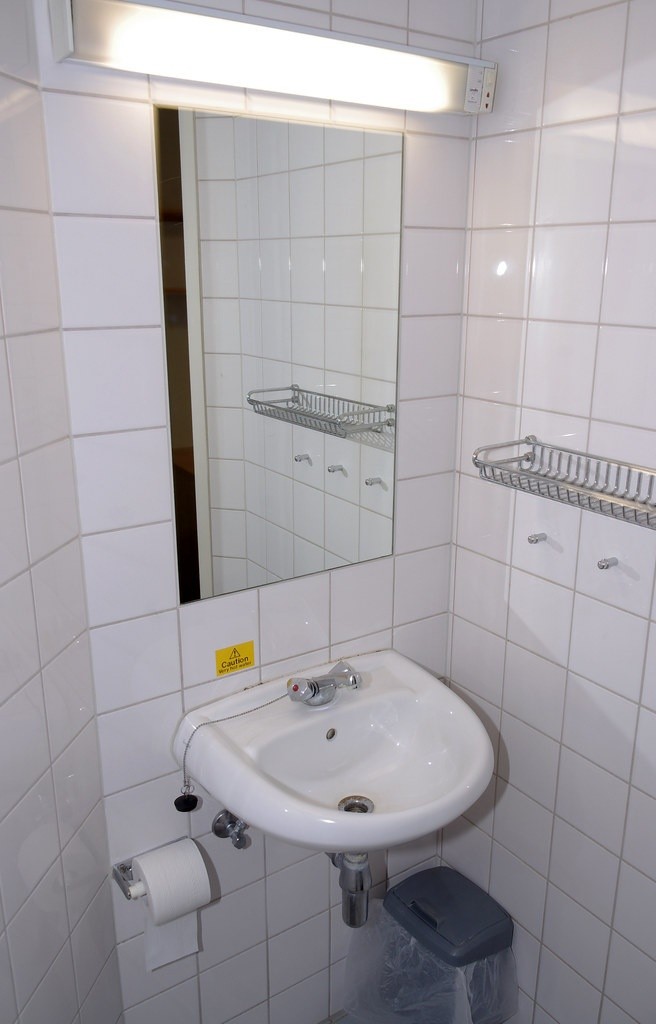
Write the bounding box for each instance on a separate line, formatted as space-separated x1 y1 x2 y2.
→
130 837 213 927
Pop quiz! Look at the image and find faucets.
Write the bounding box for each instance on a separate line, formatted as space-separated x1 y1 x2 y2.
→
286 660 361 706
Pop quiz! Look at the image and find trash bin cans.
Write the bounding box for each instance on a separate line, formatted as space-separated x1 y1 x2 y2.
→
383 865 513 1024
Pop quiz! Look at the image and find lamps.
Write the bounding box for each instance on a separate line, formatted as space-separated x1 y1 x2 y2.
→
48 0 498 115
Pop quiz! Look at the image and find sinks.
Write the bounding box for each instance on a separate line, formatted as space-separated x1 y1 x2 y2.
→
172 671 499 852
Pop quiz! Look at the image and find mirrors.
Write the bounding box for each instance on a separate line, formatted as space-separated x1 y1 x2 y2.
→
152 102 404 606
